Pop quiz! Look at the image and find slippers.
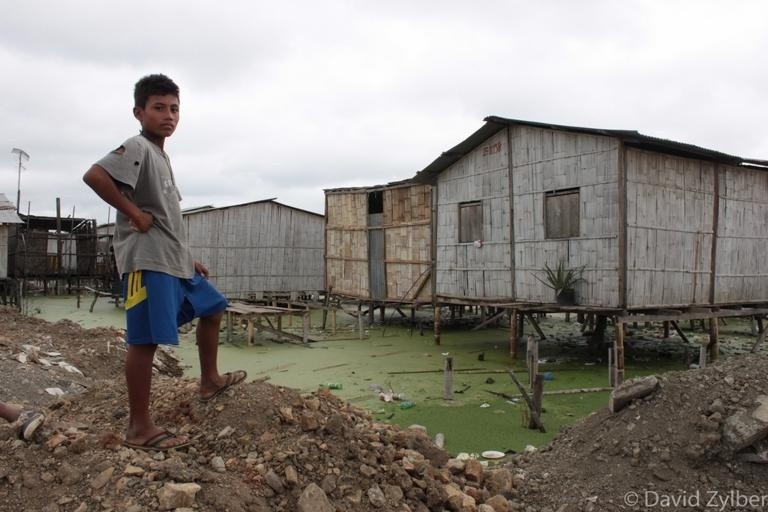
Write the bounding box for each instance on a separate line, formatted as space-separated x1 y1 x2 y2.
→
198 369 246 403
123 429 193 453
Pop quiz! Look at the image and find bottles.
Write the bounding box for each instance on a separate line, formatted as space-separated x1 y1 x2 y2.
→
317 382 342 389
392 392 416 410
435 433 445 449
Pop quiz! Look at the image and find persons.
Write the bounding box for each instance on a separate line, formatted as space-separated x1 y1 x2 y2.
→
82 72 248 450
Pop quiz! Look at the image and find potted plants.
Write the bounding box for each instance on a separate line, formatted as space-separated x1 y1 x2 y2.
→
527 258 590 308
